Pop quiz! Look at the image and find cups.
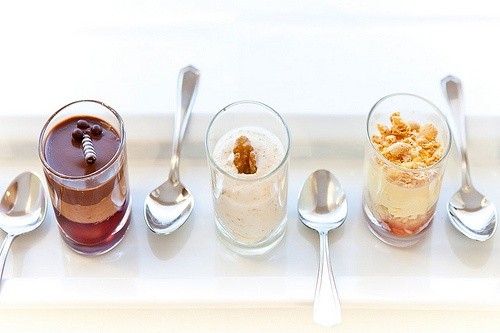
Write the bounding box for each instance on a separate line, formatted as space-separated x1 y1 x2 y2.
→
37 99 133 257
364 93 453 248
206 100 291 254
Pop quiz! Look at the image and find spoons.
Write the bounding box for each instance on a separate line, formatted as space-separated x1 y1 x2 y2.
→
0 171 47 285
298 170 348 329
441 74 496 241
144 67 200 236
